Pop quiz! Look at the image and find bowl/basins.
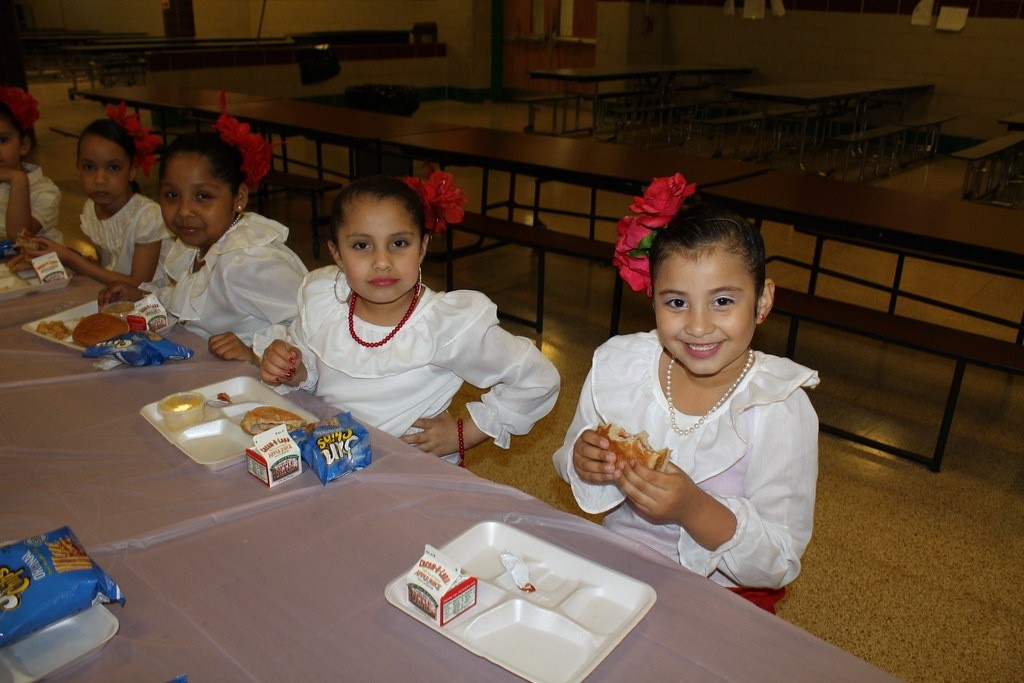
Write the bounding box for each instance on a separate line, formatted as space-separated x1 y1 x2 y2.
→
156 390 206 432
101 301 135 325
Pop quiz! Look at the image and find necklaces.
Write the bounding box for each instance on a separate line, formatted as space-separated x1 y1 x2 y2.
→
347 280 420 349
195 212 243 264
665 348 756 437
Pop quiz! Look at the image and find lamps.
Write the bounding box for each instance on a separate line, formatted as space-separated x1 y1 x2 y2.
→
935 5 969 33
721 0 787 22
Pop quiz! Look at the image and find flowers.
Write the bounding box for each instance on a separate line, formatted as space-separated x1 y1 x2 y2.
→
400 169 468 235
210 110 278 193
612 172 698 296
0 85 42 132
105 100 160 177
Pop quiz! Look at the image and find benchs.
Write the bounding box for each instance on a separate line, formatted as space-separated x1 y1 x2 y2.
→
16 83 1024 473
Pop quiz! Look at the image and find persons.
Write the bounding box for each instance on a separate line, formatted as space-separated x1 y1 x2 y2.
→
253 171 561 460
98 90 310 366
0 86 65 257
551 171 820 617
6 86 177 294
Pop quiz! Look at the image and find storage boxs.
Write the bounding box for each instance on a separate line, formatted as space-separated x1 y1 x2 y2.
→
246 424 304 489
30 250 69 284
126 296 169 334
405 541 478 629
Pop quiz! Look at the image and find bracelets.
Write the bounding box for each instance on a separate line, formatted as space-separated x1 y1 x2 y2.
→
456 417 467 461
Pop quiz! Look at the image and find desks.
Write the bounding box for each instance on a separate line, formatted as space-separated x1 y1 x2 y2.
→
75 83 284 121
380 125 770 199
726 77 936 177
0 253 900 683
697 167 1024 281
527 65 759 143
190 90 471 162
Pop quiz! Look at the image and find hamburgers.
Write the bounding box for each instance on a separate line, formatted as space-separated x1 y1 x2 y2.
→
596 421 672 474
71 312 131 346
5 254 33 272
13 228 39 249
240 406 307 435
